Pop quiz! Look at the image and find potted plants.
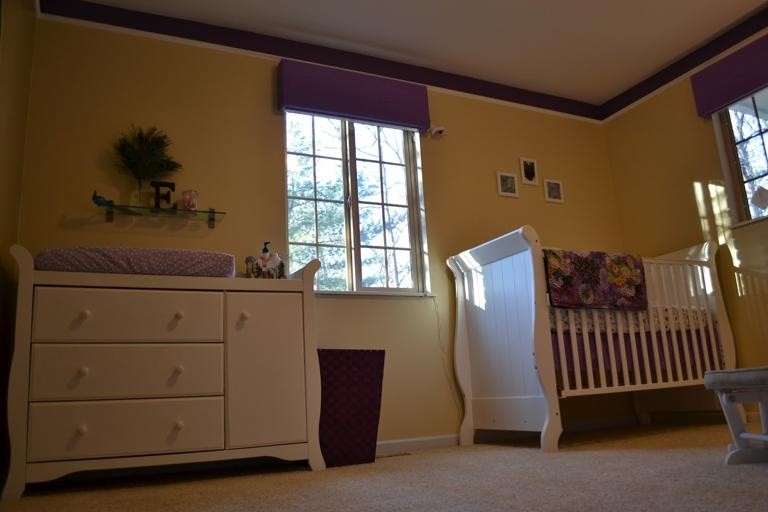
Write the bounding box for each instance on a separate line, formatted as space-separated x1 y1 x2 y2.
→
107 121 183 213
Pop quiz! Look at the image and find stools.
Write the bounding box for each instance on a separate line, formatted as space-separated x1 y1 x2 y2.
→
703 367 768 465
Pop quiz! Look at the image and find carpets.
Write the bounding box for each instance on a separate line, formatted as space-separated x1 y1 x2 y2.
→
2 443 768 511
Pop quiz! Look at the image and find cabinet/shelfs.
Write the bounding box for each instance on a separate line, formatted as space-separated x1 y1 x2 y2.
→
1 243 327 504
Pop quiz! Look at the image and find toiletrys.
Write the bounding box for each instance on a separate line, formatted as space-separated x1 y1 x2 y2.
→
263 240 271 258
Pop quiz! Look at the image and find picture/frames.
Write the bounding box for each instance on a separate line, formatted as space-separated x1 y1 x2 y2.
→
544 178 563 202
520 157 538 185
496 172 519 197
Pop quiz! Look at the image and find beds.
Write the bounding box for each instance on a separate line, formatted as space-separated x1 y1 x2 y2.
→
447 221 748 452
